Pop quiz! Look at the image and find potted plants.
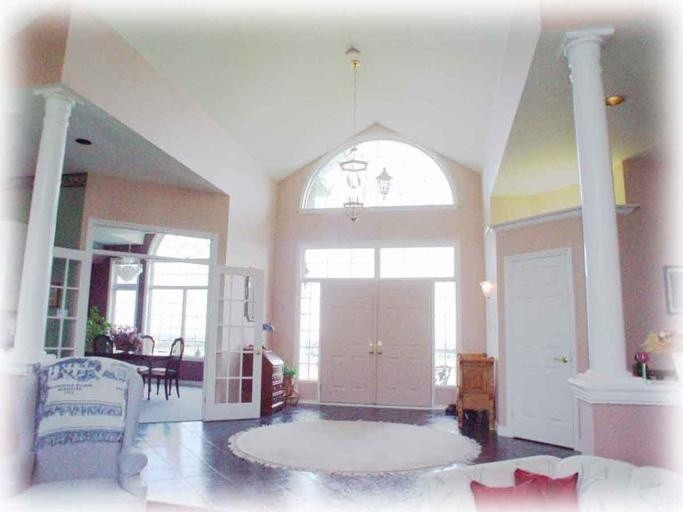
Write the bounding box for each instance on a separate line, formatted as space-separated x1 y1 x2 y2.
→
282 366 297 392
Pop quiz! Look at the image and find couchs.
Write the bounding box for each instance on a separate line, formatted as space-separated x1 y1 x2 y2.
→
413 449 680 512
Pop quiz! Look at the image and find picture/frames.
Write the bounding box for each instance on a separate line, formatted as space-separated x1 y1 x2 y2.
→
663 264 683 314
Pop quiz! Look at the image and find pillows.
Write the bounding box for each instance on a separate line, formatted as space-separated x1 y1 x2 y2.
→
468 467 580 512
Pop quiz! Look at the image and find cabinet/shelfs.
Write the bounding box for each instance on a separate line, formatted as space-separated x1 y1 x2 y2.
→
242 346 285 416
568 376 680 473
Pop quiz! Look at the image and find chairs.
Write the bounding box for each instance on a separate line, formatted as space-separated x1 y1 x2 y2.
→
4 356 150 512
93 334 185 401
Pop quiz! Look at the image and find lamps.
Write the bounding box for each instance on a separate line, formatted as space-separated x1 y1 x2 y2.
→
340 49 369 222
376 166 393 199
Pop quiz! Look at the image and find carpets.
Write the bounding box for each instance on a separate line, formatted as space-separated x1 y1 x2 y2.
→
226 421 481 474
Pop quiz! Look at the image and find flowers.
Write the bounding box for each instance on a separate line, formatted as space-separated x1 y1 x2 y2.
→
110 324 143 353
